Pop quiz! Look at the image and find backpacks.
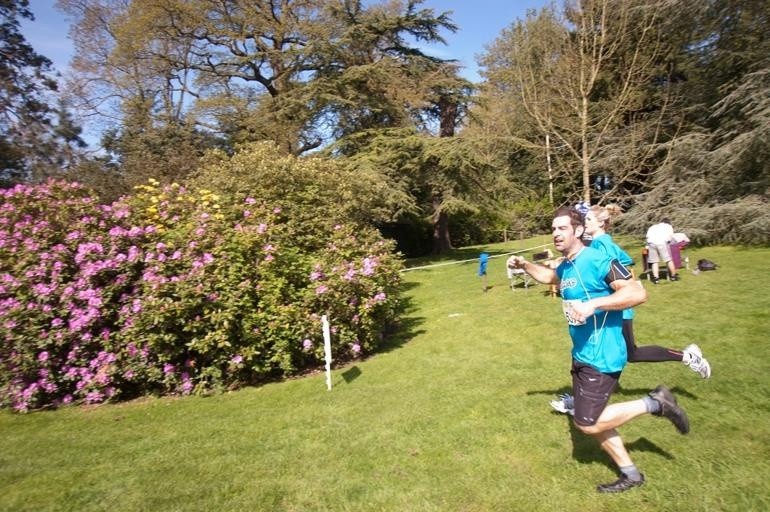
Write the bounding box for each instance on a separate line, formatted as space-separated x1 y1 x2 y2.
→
698 259 718 271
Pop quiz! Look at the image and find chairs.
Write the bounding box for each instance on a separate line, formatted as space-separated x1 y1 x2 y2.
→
505 255 540 292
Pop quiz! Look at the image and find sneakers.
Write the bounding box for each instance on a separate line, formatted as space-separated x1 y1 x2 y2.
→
548 393 576 416
684 343 713 380
595 472 645 494
653 276 660 284
649 384 691 435
670 273 680 281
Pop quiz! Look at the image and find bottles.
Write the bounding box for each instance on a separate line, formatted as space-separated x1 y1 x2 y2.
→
685 256 691 270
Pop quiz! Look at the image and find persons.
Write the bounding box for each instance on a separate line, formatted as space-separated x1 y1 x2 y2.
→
507 208 689 495
549 203 711 418
645 218 679 284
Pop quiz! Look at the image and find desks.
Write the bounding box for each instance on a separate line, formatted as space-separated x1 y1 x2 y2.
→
640 243 671 282
